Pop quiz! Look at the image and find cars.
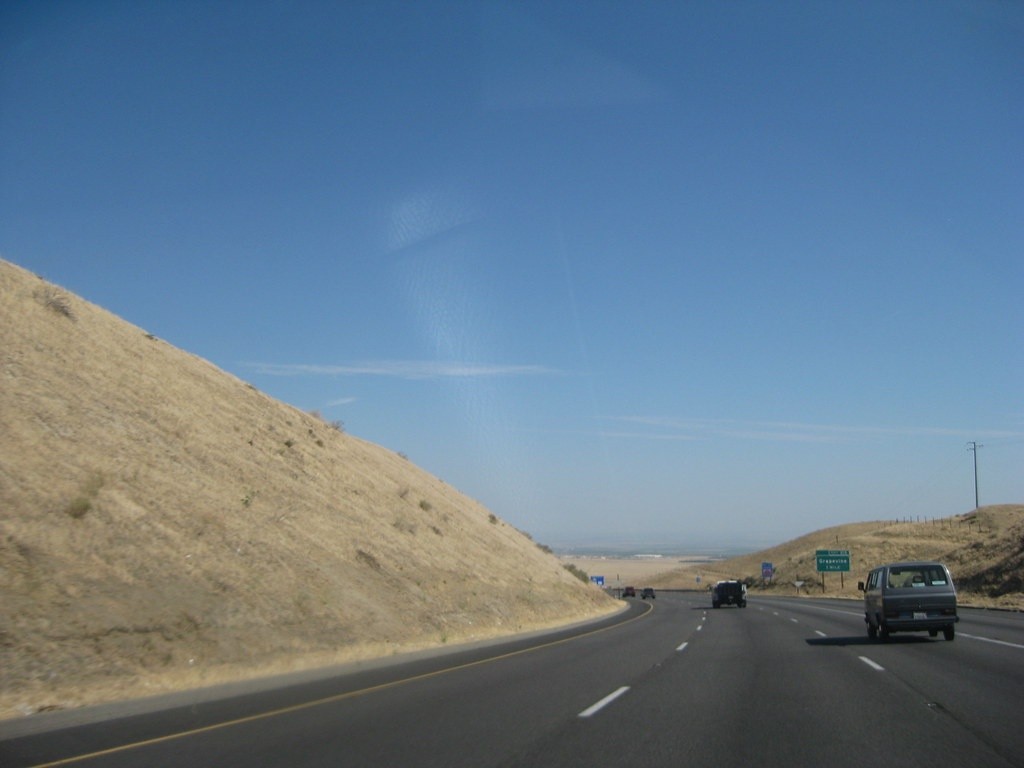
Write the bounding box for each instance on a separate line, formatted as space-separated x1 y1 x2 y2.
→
641 587 656 600
623 587 637 598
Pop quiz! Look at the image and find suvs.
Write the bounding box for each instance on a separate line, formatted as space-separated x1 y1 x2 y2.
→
858 562 959 644
711 580 747 608
